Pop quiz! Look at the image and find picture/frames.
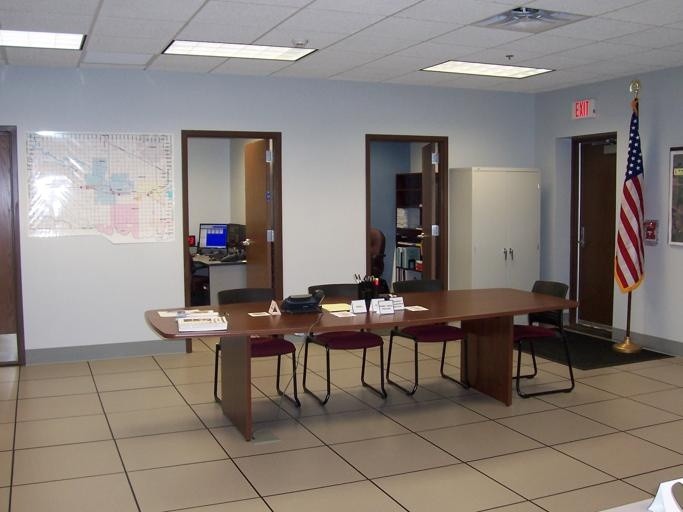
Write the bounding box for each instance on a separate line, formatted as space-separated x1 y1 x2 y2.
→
667 146 683 246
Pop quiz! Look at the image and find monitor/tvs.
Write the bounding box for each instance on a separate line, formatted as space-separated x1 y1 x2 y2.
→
199 224 227 254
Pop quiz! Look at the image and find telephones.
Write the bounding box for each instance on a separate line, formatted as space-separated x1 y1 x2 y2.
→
282 290 326 310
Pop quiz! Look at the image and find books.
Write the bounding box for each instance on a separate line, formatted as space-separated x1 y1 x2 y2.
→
176 314 228 331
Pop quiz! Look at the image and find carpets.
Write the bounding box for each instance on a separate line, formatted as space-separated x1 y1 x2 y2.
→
511 328 674 371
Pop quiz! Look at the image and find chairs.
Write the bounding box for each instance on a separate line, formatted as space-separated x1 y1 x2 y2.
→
213 287 301 409
370 228 386 277
188 253 208 306
301 283 388 405
512 280 574 398
385 279 470 396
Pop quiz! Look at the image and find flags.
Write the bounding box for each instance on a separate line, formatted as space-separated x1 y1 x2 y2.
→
614 98 644 293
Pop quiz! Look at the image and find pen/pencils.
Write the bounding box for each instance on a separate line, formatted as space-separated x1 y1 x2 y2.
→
355 274 382 288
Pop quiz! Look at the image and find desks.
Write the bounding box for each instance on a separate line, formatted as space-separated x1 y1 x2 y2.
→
192 254 247 306
143 288 581 442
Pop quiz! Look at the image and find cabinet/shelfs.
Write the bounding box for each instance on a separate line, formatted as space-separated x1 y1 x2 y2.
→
446 166 543 330
394 172 439 283
